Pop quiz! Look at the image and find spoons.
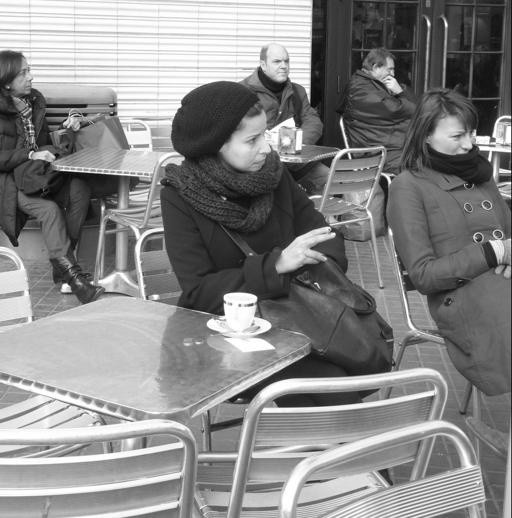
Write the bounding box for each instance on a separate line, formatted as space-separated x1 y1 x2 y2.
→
210 324 260 337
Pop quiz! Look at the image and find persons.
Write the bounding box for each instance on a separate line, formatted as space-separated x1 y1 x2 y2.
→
239 43 339 197
161 81 363 410
0 50 105 304
385 89 512 397
335 46 418 174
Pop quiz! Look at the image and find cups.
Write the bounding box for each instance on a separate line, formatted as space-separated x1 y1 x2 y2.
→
222 291 258 333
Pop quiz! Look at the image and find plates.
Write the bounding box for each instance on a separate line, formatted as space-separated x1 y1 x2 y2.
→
205 314 273 339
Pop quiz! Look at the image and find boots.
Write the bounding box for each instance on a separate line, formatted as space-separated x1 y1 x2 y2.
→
52 238 92 283
50 253 105 303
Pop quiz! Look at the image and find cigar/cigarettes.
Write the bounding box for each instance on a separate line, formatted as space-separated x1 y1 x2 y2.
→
327 228 331 234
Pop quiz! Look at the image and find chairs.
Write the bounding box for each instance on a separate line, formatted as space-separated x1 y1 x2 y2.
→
0 419 197 517
0 247 113 459
192 368 448 518
135 228 245 467
477 145 512 182
385 226 482 467
340 118 396 186
279 421 487 518
95 153 183 299
97 122 161 278
307 145 383 289
491 116 512 144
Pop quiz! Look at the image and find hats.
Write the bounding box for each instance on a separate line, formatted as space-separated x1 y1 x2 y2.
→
172 81 258 161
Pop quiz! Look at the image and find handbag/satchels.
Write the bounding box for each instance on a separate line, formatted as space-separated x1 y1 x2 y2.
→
217 220 394 375
50 108 140 197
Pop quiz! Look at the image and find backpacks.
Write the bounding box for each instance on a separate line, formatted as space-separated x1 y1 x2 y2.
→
13 145 67 196
